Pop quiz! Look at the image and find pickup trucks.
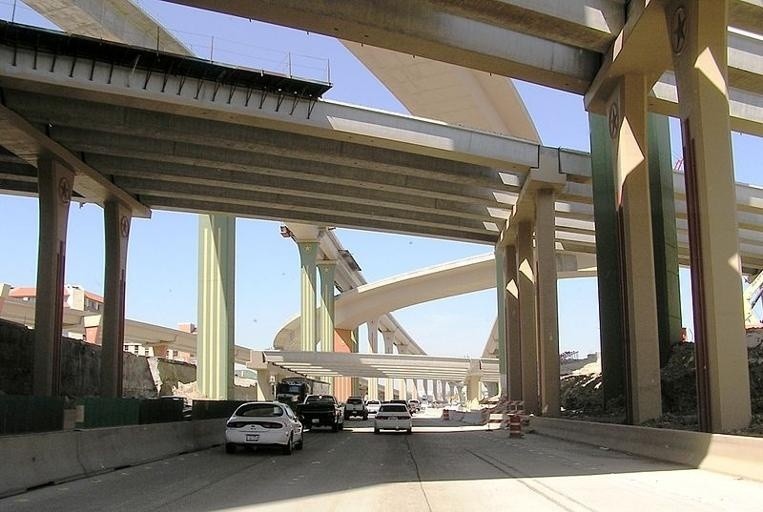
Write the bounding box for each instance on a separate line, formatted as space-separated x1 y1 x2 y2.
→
296 394 344 432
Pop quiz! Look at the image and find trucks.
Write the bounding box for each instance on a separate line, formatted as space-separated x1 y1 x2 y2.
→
277 384 301 401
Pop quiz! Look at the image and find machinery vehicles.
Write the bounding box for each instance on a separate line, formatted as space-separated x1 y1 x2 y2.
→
743 271 763 324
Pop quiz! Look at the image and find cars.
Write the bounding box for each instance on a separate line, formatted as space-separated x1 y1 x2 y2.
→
375 404 411 434
226 402 303 455
344 399 433 420
